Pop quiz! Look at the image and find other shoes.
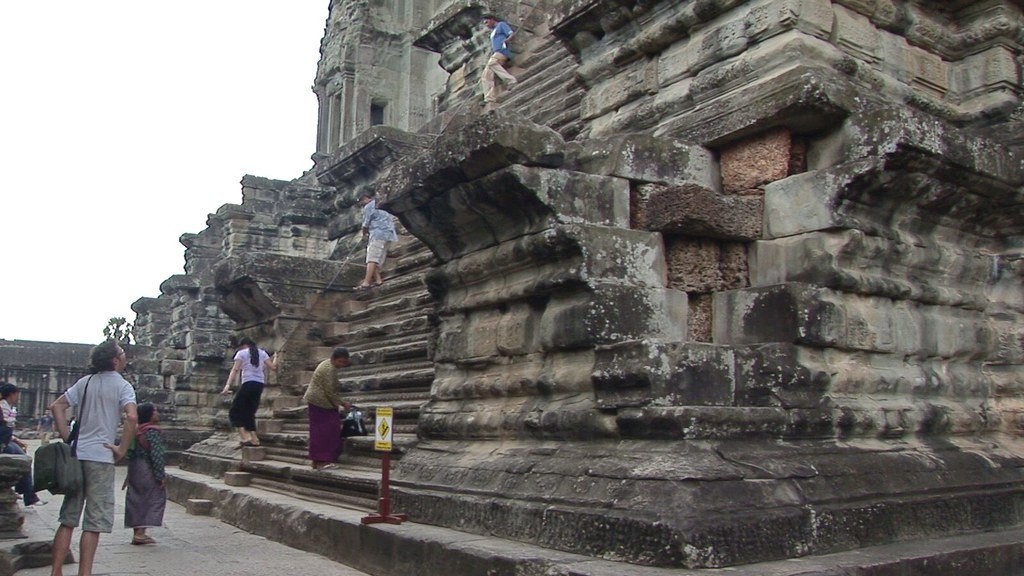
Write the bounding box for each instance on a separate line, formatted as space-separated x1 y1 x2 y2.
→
313 462 338 471
479 100 492 107
27 499 48 507
507 80 516 90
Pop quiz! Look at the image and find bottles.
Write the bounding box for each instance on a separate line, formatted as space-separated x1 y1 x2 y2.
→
8 407 17 425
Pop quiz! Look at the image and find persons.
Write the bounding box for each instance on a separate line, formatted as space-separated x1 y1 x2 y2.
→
220 336 279 450
352 193 397 290
123 402 170 545
302 347 354 471
69 416 76 432
49 338 139 576
478 14 518 107
0 383 49 507
34 408 56 446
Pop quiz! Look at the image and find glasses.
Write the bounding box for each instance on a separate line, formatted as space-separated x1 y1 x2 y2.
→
117 348 128 358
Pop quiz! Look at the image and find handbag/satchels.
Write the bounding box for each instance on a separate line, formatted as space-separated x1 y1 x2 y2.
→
31 438 83 496
342 409 368 437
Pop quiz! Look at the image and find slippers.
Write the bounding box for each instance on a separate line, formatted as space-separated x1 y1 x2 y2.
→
234 438 261 448
132 534 155 545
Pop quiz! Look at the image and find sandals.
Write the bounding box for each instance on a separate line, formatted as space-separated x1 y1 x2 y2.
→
373 282 384 288
352 285 371 292
